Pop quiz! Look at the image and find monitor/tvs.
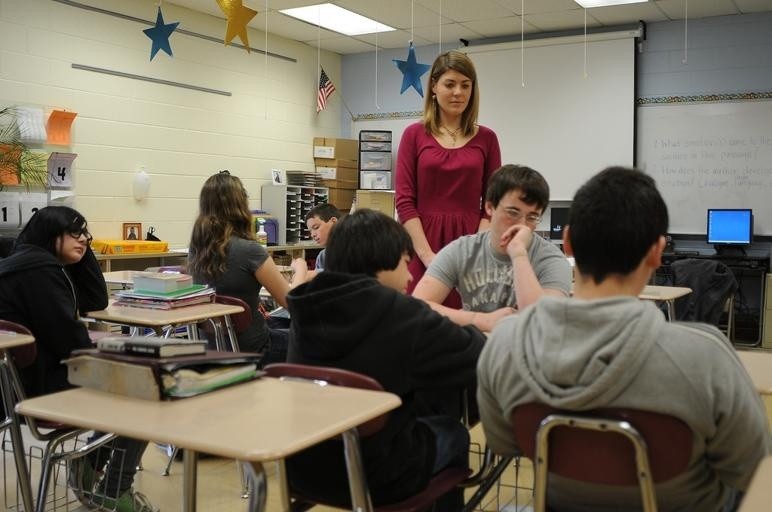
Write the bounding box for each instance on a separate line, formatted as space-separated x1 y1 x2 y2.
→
706 209 752 246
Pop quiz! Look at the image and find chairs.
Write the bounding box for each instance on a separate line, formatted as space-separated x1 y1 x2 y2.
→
248 363 473 512
0 321 122 511
510 402 693 512
672 259 735 344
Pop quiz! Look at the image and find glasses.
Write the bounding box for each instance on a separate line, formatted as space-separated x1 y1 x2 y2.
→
498 202 544 226
69 224 93 245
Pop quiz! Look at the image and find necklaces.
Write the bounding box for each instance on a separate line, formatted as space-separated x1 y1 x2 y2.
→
439 122 463 147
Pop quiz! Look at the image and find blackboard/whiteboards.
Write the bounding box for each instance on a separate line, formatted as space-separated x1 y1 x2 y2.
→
351 111 427 192
634 90 772 240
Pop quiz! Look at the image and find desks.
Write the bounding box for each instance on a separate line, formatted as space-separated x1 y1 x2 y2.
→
0 329 36 512
570 287 692 323
14 377 402 512
655 254 768 347
95 245 326 272
85 295 246 339
481 328 771 400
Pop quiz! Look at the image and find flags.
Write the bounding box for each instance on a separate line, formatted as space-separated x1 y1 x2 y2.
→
316 70 336 114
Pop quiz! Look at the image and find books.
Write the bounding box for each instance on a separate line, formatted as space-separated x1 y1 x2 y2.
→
97 335 208 358
111 271 216 310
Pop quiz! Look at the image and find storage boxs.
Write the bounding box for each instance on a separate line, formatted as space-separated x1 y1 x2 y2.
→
314 159 358 191
327 188 355 210
324 137 360 161
313 138 325 159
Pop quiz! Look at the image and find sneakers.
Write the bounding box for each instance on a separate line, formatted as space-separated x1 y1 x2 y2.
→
66 455 104 509
89 486 160 512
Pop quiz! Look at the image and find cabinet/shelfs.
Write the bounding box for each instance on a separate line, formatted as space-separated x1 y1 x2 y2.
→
356 190 395 219
261 185 330 246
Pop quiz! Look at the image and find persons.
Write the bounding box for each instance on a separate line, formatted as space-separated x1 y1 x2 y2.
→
0 205 151 511
128 226 136 239
411 164 584 332
187 173 307 366
395 51 502 310
285 209 488 511
306 203 344 269
478 164 769 512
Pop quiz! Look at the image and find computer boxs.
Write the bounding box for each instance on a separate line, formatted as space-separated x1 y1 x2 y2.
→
761 273 771 350
